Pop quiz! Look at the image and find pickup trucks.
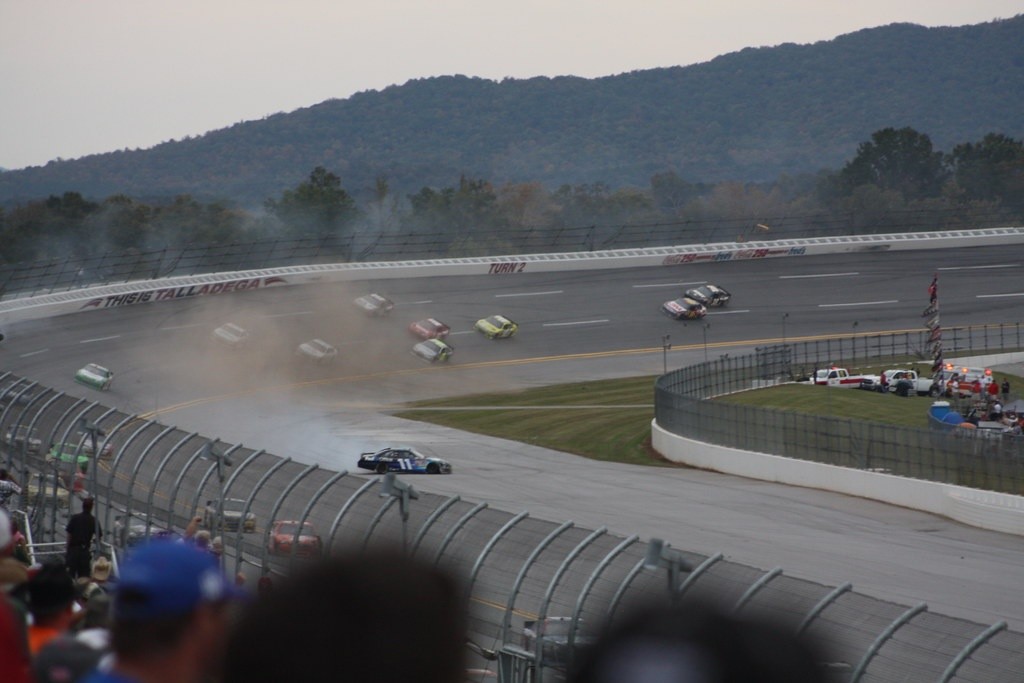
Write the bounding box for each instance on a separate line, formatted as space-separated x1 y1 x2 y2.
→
809 367 875 391
862 369 935 396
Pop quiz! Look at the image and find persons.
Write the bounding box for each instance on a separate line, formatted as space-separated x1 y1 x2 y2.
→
1001 378 1010 405
987 380 999 401
0 472 830 682
952 377 959 400
880 371 886 391
973 382 981 400
993 403 1002 418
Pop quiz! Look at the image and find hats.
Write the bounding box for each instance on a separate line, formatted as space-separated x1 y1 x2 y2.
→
196 530 211 545
211 535 225 553
112 538 254 627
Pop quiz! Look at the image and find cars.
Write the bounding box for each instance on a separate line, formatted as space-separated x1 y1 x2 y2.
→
77 429 113 456
662 296 707 320
411 317 450 341
298 338 338 360
354 293 394 316
412 338 455 363
212 322 249 348
75 363 114 393
205 498 257 533
5 423 41 453
684 284 731 308
473 315 516 340
357 446 452 475
934 364 996 398
270 519 321 554
49 443 91 474
